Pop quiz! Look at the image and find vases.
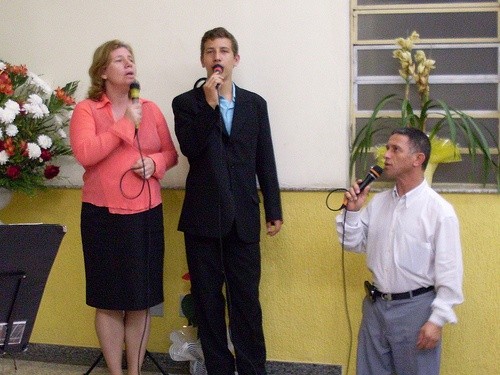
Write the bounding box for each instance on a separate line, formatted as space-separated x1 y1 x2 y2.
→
375 133 462 188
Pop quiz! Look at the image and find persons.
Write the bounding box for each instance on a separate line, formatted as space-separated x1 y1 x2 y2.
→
171 26 284 375
335 127 465 375
70 40 179 375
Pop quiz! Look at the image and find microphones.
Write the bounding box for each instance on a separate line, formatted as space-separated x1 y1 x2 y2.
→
213 64 223 89
130 83 140 133
342 165 383 210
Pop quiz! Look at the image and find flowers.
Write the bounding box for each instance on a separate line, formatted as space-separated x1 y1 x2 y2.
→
351 31 500 194
0 58 79 200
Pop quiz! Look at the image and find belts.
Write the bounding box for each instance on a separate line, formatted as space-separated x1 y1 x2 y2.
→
371 285 435 300
